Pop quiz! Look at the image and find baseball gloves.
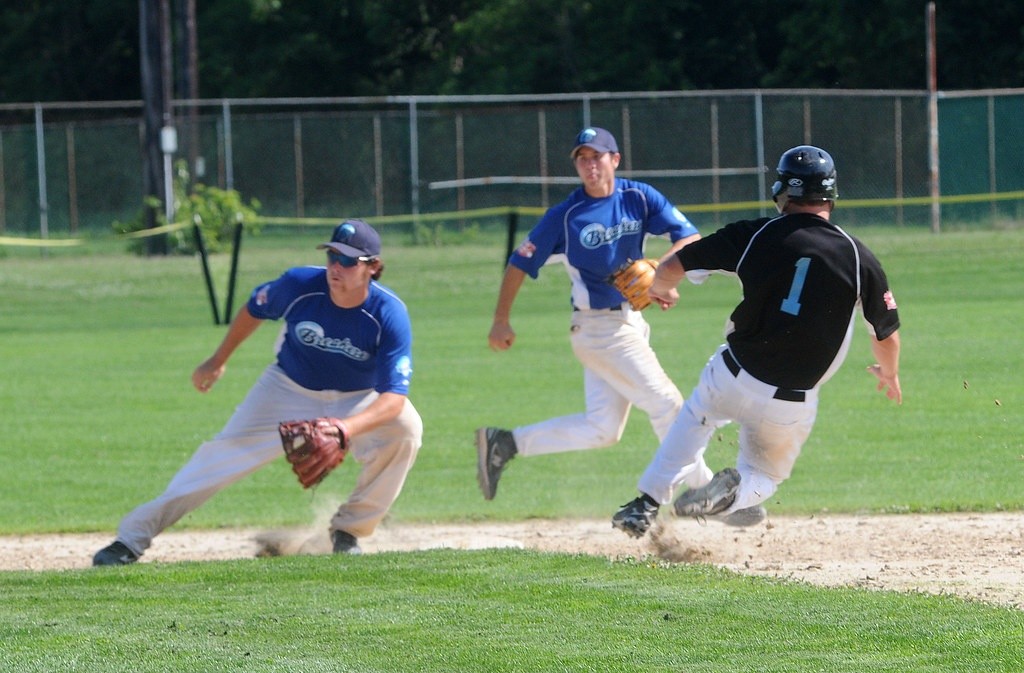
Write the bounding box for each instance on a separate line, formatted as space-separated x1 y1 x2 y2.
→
609 257 660 312
279 416 351 490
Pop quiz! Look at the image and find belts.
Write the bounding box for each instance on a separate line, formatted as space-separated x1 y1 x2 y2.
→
573 303 622 310
722 350 806 402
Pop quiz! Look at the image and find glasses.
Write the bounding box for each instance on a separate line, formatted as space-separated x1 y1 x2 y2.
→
326 250 377 267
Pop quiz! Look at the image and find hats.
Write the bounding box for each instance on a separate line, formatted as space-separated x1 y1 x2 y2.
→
570 127 618 160
316 219 381 258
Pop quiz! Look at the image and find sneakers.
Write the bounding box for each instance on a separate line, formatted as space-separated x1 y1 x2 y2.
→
330 529 361 554
721 505 766 527
674 468 742 526
93 542 137 567
474 428 517 499
612 498 658 539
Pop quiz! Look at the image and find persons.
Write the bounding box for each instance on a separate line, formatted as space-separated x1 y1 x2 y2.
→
612 146 902 540
94 218 423 565
477 126 766 527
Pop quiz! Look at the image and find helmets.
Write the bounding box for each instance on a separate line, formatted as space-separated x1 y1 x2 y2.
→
774 145 839 201
